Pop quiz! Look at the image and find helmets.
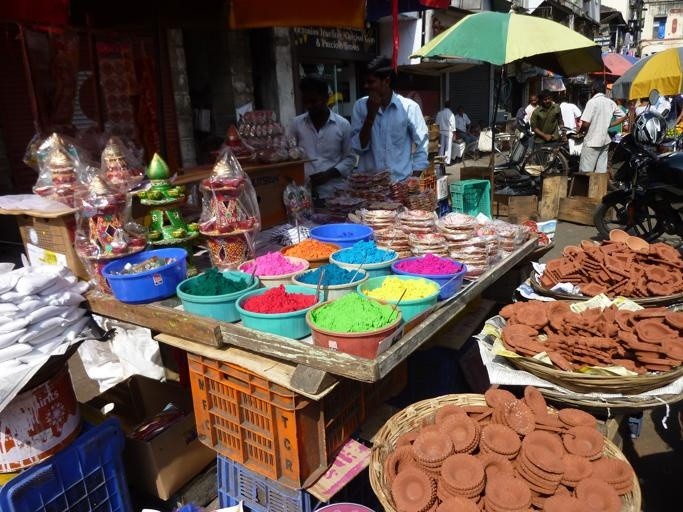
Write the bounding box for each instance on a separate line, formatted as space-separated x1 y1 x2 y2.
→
631 111 667 147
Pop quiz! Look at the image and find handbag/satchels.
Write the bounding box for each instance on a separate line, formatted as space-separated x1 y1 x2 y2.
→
477 127 492 152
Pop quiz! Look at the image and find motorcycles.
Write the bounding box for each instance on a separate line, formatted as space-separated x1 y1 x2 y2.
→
492 89 683 245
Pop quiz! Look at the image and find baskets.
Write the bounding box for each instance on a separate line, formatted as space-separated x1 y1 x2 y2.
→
529 236 682 306
369 393 641 512
188 344 641 512
486 297 681 392
0 416 130 512
419 174 490 219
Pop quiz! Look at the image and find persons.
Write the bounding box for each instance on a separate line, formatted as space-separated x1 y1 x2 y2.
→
518 80 683 171
351 55 431 182
287 78 353 209
434 100 457 165
455 105 479 153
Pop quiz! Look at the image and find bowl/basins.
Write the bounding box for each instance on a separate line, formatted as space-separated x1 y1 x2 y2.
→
103 247 189 303
392 256 466 300
304 298 404 361
354 275 441 324
279 241 342 269
238 255 312 277
308 224 374 248
562 227 649 256
293 268 370 301
327 247 400 280
175 268 259 324
240 284 324 342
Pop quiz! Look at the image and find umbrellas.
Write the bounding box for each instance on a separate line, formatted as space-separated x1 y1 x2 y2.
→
407 8 606 133
612 47 683 146
589 53 639 76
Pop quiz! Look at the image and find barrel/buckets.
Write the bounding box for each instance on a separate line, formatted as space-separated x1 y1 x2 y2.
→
0 364 82 475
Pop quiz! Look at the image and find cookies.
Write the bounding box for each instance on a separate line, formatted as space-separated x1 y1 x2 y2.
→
538 229 683 300
498 299 683 376
384 384 634 512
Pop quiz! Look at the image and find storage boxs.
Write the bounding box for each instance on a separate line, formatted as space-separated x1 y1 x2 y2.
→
558 195 613 226
16 196 147 282
460 165 492 181
492 194 538 216
80 332 194 421
248 156 307 230
436 175 448 200
78 403 216 502
568 172 608 199
538 173 567 221
435 297 497 393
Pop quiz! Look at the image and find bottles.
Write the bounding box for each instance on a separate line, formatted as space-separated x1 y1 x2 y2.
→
627 411 644 440
